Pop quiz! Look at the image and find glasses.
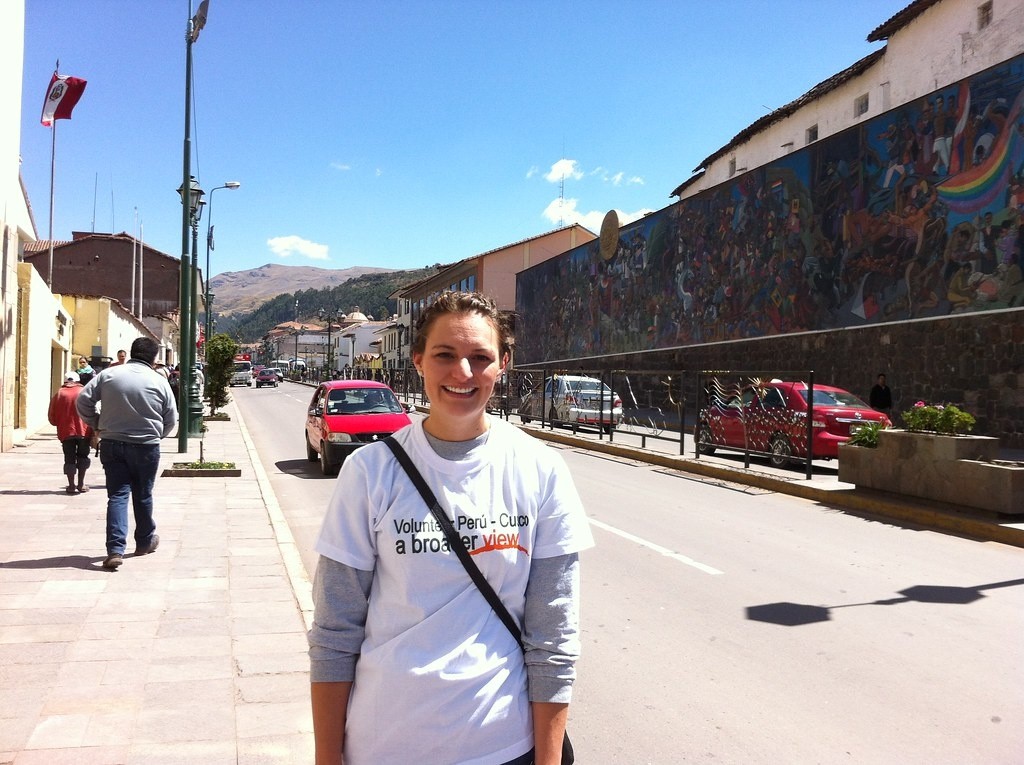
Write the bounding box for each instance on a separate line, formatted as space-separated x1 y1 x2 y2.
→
79 362 85 364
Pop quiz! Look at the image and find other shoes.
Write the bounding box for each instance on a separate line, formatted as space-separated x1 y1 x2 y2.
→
66 487 78 494
134 535 159 556
79 486 88 492
103 554 122 571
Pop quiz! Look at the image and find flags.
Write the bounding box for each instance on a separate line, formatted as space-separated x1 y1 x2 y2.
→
40 71 87 126
196 325 203 348
196 322 200 343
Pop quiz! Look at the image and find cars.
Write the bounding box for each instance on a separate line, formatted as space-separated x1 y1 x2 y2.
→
305 380 417 476
693 383 892 469
518 376 623 433
487 372 514 416
267 367 284 382
256 368 279 388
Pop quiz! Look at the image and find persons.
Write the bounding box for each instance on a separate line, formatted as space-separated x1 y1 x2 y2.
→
870 374 892 417
332 365 419 392
308 290 595 765
154 363 174 378
48 372 93 494
110 350 126 366
301 365 306 382
345 363 354 375
76 356 96 385
76 337 176 569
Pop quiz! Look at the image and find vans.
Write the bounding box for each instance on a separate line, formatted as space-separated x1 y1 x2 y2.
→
271 359 291 378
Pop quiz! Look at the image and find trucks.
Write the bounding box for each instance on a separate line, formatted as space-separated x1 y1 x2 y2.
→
228 361 254 387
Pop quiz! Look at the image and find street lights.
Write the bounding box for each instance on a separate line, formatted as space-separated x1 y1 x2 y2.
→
174 174 206 438
210 318 217 338
351 335 357 370
396 323 406 368
318 304 343 382
287 324 306 381
207 287 215 338
205 179 241 363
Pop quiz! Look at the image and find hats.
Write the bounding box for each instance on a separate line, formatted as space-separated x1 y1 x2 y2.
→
155 360 165 366
64 371 81 382
